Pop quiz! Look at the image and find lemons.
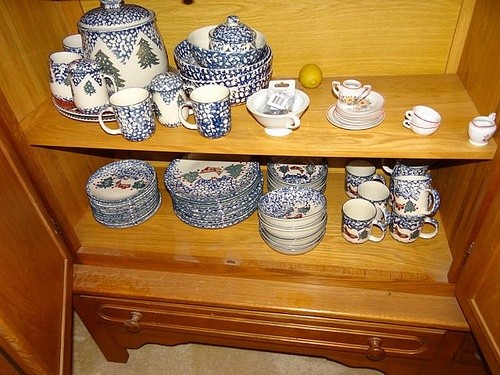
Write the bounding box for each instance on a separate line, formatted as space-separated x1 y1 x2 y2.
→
299 64 322 88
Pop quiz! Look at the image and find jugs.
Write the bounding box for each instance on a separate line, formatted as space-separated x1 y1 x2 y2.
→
468 112 497 147
76 0 170 96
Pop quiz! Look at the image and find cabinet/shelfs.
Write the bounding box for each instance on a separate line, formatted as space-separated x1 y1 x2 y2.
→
0 0 500 375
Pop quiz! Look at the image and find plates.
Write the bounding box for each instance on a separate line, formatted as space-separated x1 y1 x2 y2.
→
85 159 162 229
267 157 329 194
50 94 155 123
164 152 264 228
326 90 385 130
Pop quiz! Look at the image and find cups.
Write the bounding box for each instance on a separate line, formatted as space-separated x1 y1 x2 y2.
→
402 104 442 135
343 160 386 199
48 51 83 103
331 79 372 109
383 159 441 243
178 85 232 140
97 87 157 142
340 198 386 244
63 58 118 116
61 35 83 57
207 15 257 53
357 180 391 227
149 72 195 129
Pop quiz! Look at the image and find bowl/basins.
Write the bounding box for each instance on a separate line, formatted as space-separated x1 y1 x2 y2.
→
256 186 328 255
172 26 273 107
246 86 310 137
